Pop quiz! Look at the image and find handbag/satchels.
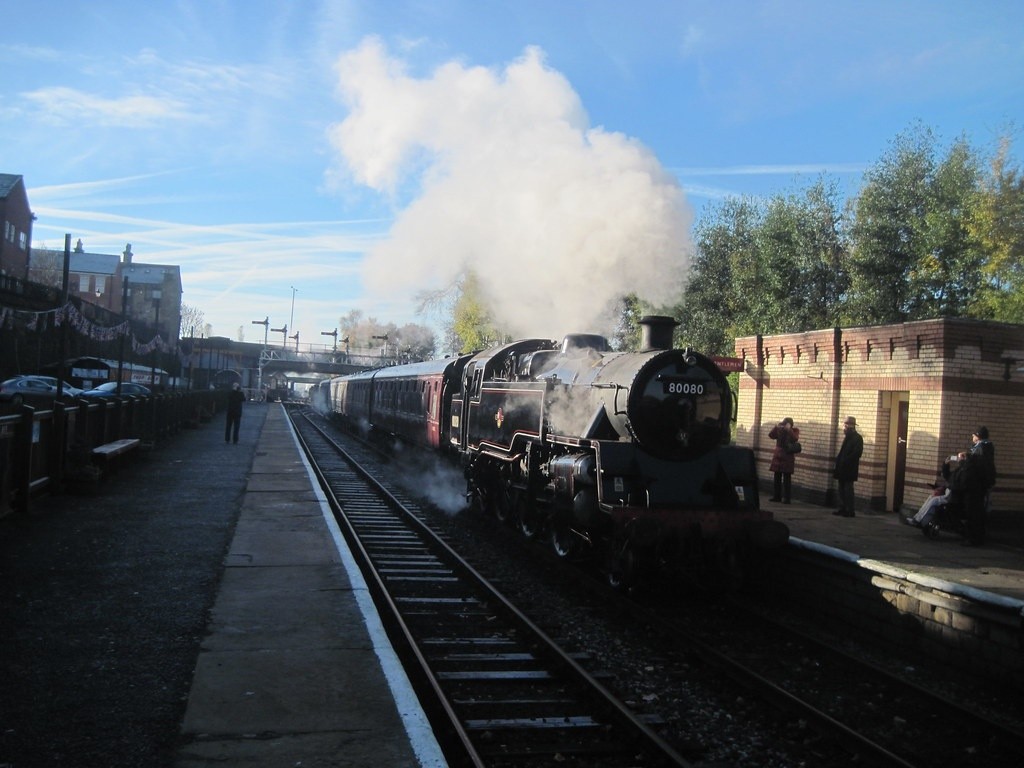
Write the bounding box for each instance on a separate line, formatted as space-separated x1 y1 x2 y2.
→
786 443 801 454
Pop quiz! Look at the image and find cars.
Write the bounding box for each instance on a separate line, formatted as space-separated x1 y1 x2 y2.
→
78 382 151 404
0 378 74 409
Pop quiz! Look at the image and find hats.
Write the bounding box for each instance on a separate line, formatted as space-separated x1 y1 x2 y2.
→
845 417 858 425
778 418 793 425
232 383 240 390
973 426 989 439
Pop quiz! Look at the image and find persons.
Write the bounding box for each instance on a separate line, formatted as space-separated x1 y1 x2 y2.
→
832 417 863 518
225 383 246 444
904 426 997 546
768 418 799 504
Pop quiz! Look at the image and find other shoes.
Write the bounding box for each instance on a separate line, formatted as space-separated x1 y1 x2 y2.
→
782 501 791 504
769 499 781 502
906 518 920 526
833 510 855 517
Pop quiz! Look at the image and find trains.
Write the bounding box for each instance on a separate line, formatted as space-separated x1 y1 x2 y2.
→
308 315 790 606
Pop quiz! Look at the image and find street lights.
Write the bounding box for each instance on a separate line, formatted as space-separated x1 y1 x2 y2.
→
288 285 298 357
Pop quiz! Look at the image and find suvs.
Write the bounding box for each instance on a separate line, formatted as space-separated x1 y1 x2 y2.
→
17 375 85 401
